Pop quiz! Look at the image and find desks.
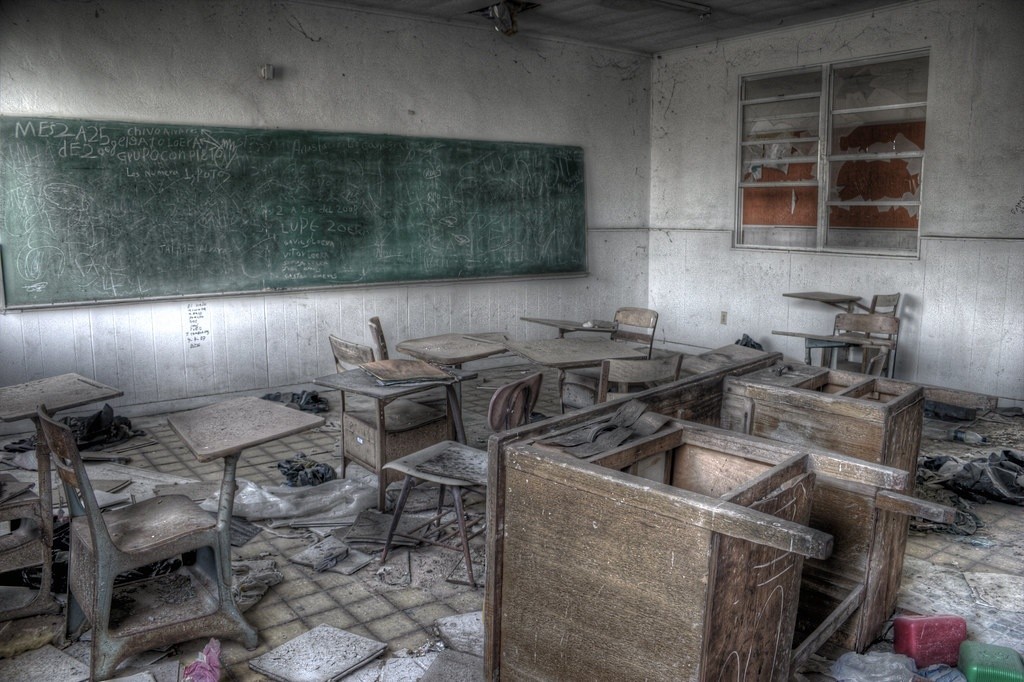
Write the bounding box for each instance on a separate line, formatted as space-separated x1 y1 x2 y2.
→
519 315 618 339
476 353 956 682
399 333 509 368
770 330 874 372
0 371 123 497
314 360 479 525
781 290 866 318
507 337 648 370
167 396 325 524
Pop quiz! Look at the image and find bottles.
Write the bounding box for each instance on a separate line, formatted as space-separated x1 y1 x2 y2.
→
948 429 986 444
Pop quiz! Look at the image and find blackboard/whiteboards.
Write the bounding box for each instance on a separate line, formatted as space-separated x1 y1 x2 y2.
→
0 114 590 315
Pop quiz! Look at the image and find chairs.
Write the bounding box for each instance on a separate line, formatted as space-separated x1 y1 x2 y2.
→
327 333 375 373
610 303 659 391
38 404 259 682
382 369 545 588
599 350 686 406
832 317 907 379
367 315 387 358
826 290 901 372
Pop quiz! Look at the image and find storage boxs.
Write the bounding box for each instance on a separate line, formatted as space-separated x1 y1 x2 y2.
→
893 613 966 669
958 640 1024 682
